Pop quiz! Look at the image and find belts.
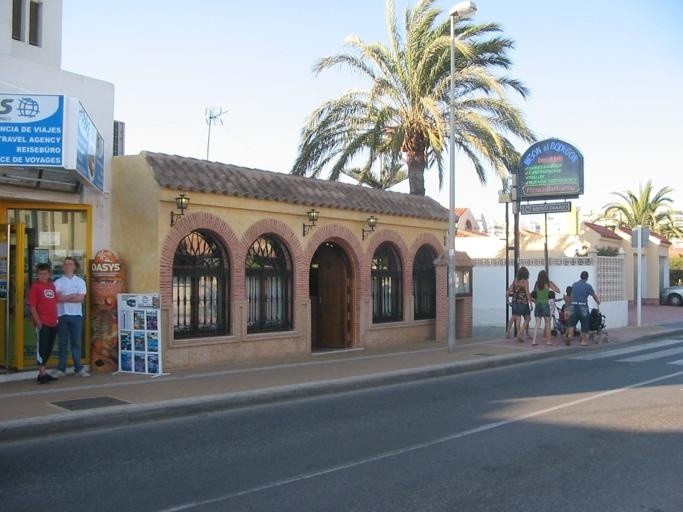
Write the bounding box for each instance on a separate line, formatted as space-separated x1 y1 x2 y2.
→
571 302 587 305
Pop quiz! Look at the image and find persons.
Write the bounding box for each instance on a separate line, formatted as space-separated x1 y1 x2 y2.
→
53 256 91 379
25 262 59 385
511 267 533 343
529 269 560 345
562 271 600 346
546 290 563 330
501 279 533 340
563 286 572 307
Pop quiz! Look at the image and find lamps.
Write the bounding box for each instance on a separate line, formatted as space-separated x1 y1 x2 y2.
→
303 208 320 237
361 215 380 241
169 193 191 227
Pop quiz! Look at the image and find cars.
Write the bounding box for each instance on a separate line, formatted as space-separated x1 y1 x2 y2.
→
660 282 683 307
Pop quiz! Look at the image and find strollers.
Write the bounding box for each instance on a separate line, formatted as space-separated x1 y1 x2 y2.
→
588 301 612 347
551 293 581 339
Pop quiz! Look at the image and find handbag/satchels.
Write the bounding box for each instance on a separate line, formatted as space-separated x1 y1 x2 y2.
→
530 291 537 299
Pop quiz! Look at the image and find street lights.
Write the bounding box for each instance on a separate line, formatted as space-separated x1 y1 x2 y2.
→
442 1 481 355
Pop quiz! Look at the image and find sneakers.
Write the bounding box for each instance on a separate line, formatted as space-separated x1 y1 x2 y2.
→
36 367 90 384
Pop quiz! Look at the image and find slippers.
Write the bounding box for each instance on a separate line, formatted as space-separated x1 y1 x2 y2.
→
503 331 590 347
